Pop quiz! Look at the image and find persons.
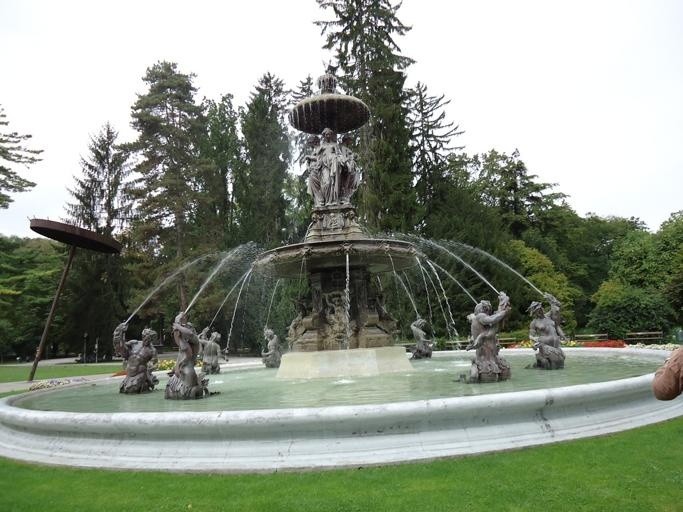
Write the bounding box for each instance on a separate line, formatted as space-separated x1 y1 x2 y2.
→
524 291 566 371
650 343 682 402
404 315 435 361
196 327 228 376
314 126 347 209
161 310 213 400
111 320 160 395
296 135 320 211
454 291 511 385
338 132 361 204
283 296 306 352
367 291 401 334
320 288 352 342
260 327 283 371
